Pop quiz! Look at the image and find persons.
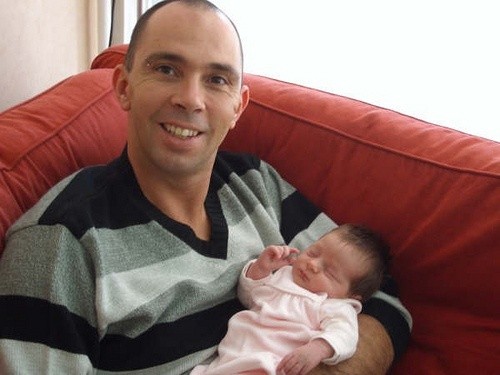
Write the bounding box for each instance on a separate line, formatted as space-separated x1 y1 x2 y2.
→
182 222 393 375
0 0 416 375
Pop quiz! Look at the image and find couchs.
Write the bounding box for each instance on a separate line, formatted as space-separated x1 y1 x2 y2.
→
0 44 500 375
